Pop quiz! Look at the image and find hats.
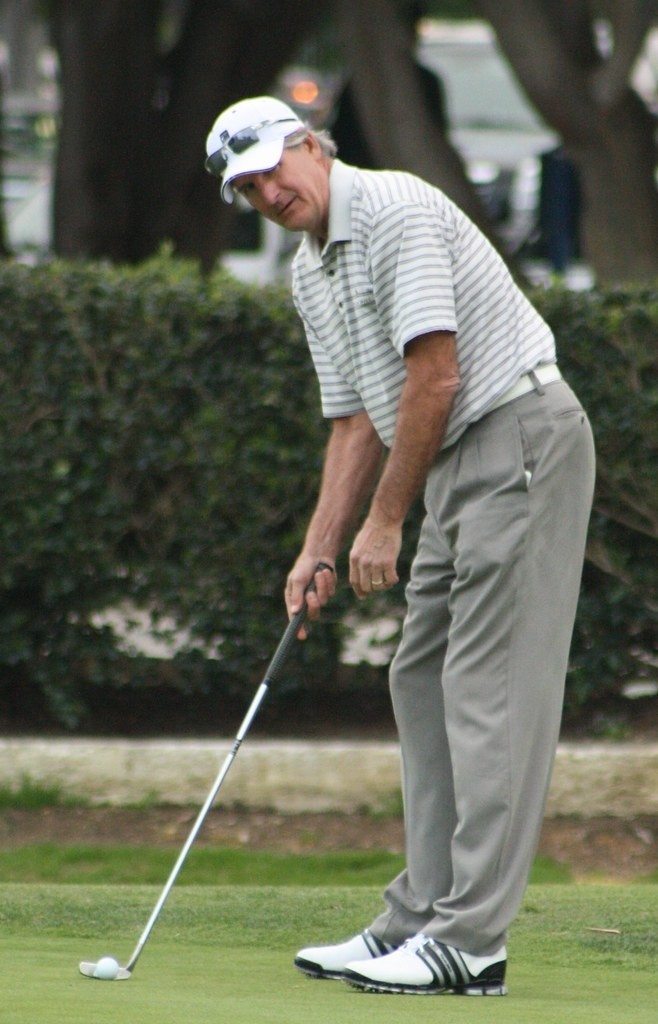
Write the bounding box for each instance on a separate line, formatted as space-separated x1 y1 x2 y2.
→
205 96 306 205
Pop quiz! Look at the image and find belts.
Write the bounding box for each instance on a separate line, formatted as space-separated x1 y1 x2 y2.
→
491 366 563 408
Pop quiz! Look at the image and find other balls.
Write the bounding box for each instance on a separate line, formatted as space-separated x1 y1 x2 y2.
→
96 957 119 980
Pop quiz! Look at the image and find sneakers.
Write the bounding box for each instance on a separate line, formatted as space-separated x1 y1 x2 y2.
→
340 933 507 997
294 929 397 978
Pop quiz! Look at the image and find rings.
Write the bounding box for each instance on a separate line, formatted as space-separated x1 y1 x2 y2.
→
372 578 385 587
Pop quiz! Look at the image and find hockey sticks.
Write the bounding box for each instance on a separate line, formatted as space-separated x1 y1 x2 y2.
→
77 560 331 982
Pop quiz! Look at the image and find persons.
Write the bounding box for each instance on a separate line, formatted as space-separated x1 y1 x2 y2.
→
204 96 594 996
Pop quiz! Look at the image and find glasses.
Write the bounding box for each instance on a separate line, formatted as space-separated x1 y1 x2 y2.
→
205 116 299 177
